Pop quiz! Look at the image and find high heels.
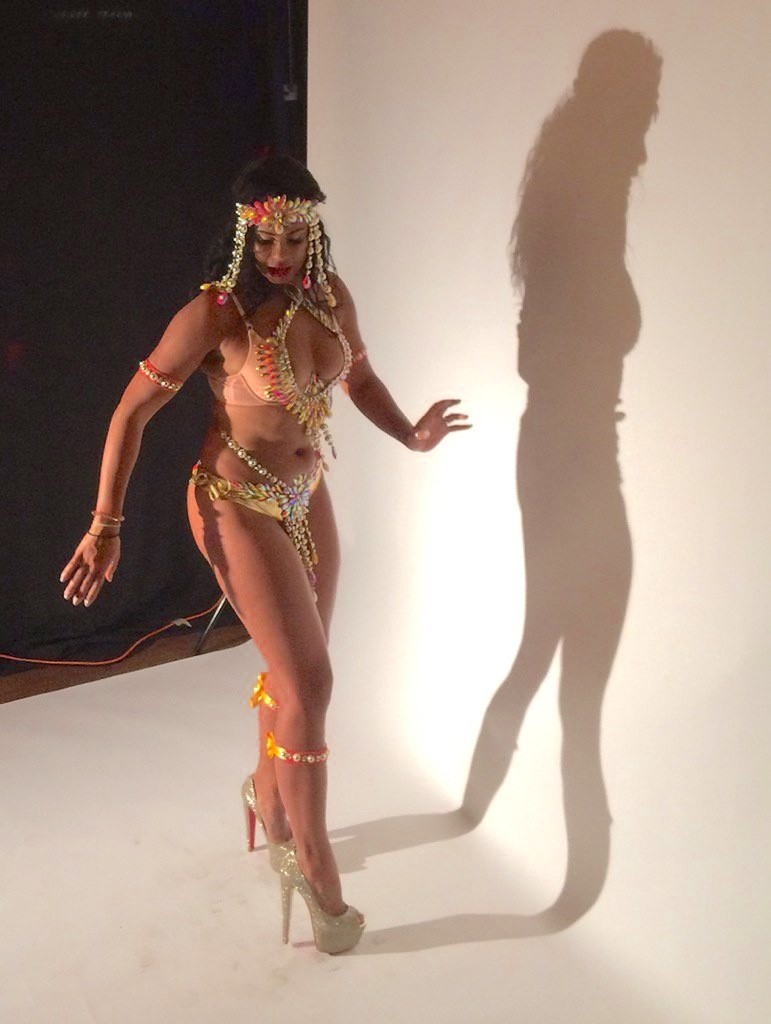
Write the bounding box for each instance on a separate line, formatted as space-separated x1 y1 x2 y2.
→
270 841 366 955
242 775 297 872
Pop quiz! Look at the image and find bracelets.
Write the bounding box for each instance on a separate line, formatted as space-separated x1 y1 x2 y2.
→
92 520 121 527
87 530 119 538
92 511 124 521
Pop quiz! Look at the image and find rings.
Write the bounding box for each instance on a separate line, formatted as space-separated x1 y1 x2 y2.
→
70 560 80 568
445 417 449 423
90 567 98 573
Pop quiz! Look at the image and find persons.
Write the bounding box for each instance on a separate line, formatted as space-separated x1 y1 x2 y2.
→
59 147 474 953
